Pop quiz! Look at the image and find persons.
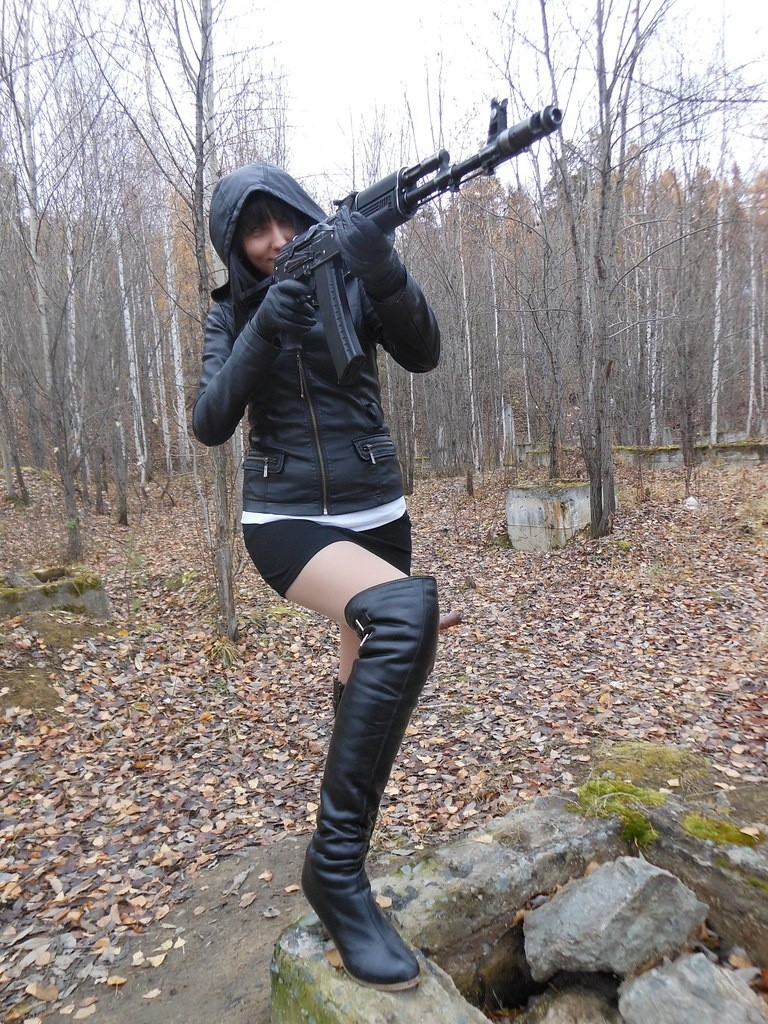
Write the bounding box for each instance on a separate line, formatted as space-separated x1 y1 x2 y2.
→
191 163 441 993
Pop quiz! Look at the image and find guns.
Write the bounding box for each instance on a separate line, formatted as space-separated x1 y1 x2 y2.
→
273 95 566 389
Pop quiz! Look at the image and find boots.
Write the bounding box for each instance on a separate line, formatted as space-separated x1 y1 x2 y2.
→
301 575 439 990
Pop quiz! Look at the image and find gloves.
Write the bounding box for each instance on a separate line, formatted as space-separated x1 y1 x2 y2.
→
250 278 317 342
334 205 407 299
